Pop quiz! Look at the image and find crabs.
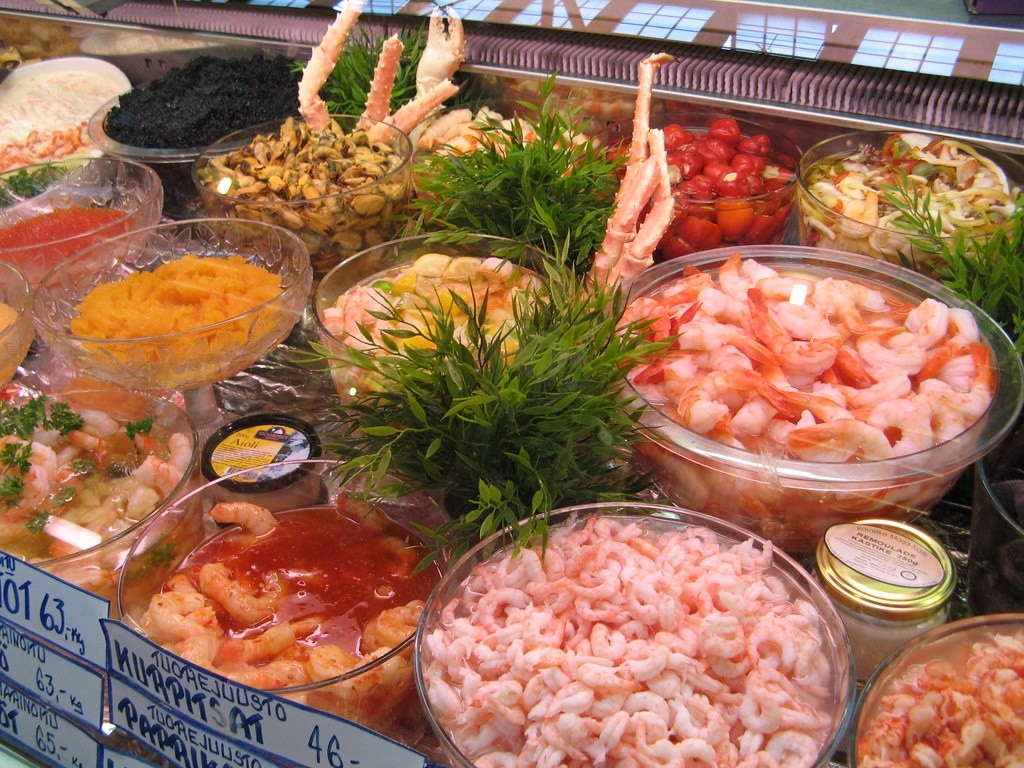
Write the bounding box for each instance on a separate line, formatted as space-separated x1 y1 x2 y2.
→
579 52 676 321
296 0 467 148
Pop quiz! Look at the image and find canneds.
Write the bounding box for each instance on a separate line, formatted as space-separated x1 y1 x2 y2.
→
810 518 958 763
200 414 321 512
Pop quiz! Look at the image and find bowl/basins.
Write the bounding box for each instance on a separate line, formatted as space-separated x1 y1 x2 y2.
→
0 259 33 386
407 97 603 217
190 113 413 280
114 457 457 751
0 154 164 294
580 112 804 262
605 242 1024 553
411 500 847 768
0 388 203 617
795 128 1024 281
845 613 1024 768
29 218 312 388
86 80 267 212
312 230 575 450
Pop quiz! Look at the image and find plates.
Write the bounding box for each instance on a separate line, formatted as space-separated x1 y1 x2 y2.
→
0 57 133 182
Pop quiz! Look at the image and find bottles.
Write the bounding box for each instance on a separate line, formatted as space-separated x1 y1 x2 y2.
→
805 516 958 768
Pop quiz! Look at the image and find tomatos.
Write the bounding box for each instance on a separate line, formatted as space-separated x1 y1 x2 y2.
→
607 119 796 259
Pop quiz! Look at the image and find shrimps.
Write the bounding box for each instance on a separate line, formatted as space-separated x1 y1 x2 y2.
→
0 249 1024 768
797 131 1022 280
412 107 602 221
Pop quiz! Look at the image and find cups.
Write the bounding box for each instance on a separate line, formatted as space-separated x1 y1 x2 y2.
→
968 400 1024 619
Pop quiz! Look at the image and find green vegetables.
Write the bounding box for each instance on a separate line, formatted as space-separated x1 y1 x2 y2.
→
0 395 152 530
